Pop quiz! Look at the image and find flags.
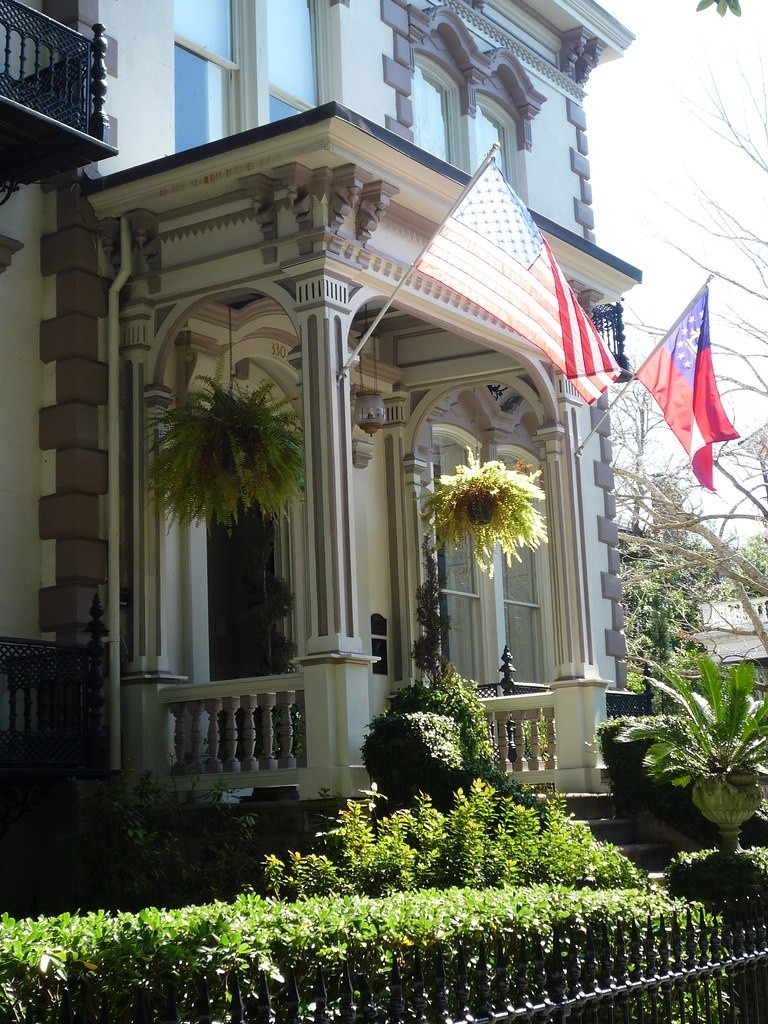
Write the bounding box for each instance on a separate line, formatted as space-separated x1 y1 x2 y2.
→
415 155 622 406
636 286 740 492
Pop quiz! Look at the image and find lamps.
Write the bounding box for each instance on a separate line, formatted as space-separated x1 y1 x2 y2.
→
354 302 386 437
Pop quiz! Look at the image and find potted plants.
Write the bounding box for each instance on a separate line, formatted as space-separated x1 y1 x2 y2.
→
411 445 550 580
138 376 309 539
613 655 768 855
